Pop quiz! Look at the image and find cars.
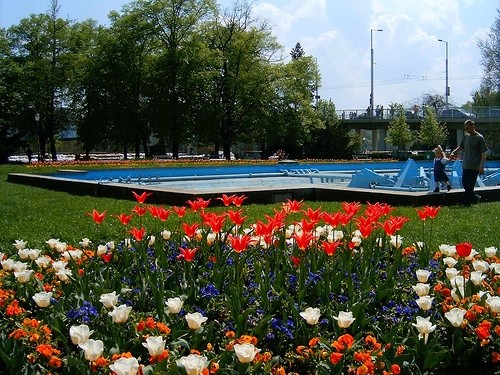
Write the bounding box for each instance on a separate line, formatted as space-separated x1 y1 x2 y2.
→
357 113 383 119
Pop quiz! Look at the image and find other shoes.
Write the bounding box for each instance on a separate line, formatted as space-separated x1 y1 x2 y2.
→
434 187 440 193
472 195 482 206
447 185 452 191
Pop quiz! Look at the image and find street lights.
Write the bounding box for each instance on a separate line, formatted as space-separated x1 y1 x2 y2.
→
369 28 384 119
438 39 449 117
35 112 40 163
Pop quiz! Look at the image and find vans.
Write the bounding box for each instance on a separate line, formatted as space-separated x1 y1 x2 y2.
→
441 108 476 118
392 110 423 119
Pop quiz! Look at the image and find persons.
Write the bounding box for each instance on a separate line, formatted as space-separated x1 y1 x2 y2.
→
449 120 488 206
413 105 418 114
390 106 394 115
433 145 453 192
376 105 380 116
